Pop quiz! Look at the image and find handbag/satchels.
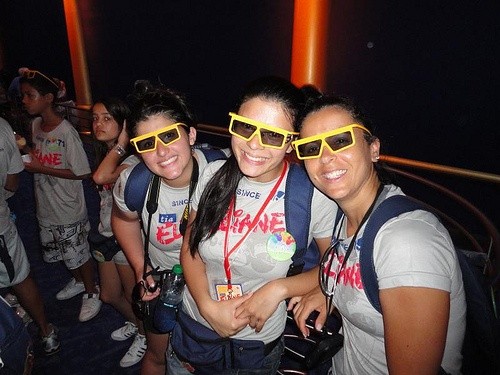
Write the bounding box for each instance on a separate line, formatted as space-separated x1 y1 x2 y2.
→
87 225 121 263
131 265 185 334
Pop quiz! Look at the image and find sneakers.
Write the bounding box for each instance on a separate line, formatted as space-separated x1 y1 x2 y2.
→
15 306 33 327
120 332 147 367
79 285 103 322
56 277 86 300
111 321 139 341
39 323 62 357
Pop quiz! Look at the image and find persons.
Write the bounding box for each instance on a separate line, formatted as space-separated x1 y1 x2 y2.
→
0 116 61 354
110 75 231 375
166 80 339 375
15 69 102 321
91 96 148 368
287 93 466 375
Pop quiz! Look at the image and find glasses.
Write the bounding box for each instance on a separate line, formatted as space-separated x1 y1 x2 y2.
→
129 122 189 154
18 68 58 88
291 124 373 161
228 111 300 151
318 239 343 297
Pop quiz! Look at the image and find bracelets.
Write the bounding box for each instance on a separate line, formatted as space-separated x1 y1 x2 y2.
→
113 144 126 156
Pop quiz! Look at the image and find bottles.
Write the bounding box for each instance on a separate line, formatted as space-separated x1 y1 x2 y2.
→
159 265 185 307
14 134 32 162
